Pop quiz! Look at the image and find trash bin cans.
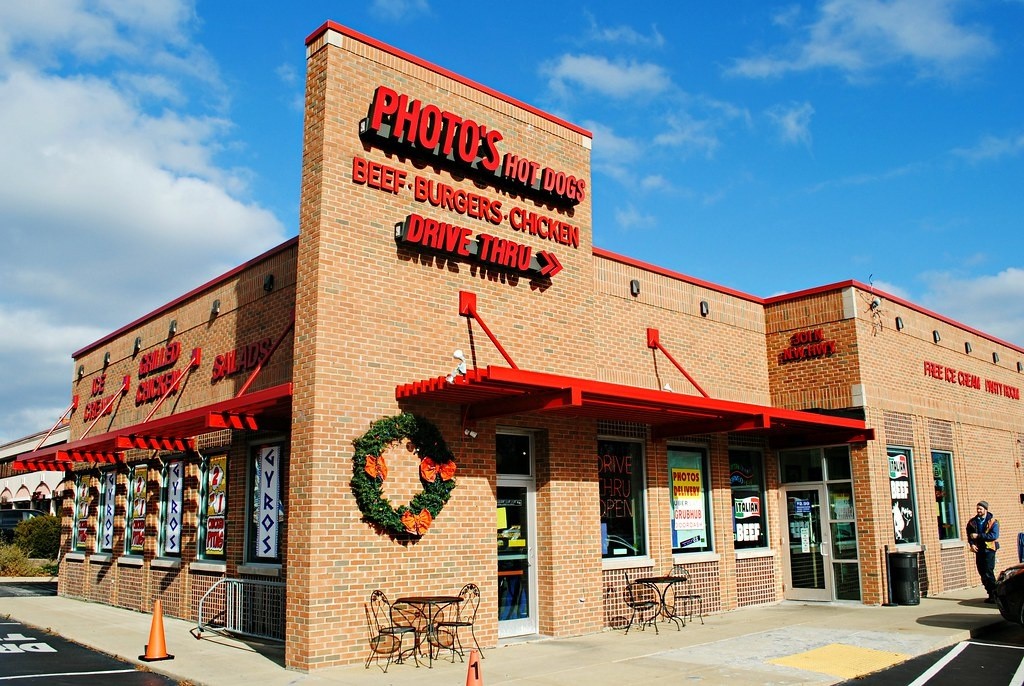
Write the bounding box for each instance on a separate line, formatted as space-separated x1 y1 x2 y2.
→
889 551 920 605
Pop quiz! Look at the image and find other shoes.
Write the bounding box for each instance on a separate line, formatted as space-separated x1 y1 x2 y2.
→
984 598 996 604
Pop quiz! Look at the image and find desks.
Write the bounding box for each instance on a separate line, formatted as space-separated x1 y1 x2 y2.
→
498 570 523 576
635 577 688 632
397 596 465 669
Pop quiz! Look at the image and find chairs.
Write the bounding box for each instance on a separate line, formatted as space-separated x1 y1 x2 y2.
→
365 590 420 674
668 566 705 626
624 572 660 636
434 583 486 663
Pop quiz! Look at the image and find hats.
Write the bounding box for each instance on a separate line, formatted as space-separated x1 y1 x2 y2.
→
977 501 988 510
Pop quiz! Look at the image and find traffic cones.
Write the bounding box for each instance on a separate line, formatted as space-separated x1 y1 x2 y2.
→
465 650 484 686
138 599 174 662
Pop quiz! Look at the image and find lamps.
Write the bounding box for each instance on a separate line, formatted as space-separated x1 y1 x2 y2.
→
1017 362 1024 371
965 342 972 352
104 352 111 364
631 280 640 294
169 320 177 333
701 301 709 315
993 352 1000 362
135 337 141 349
933 330 941 341
263 274 274 292
211 299 220 315
896 317 904 329
77 365 84 375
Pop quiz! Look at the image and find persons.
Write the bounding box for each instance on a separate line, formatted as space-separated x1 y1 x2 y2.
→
966 501 1000 603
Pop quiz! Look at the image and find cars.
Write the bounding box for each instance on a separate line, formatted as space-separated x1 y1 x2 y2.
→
0 509 50 543
993 562 1024 623
